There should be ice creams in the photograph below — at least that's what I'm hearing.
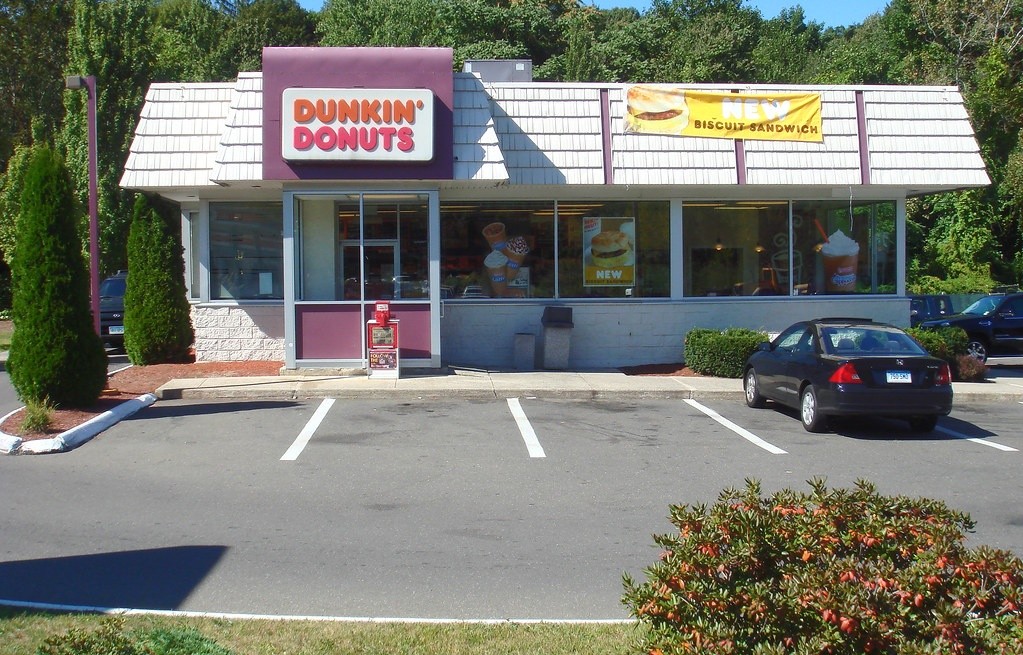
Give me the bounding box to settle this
[482,222,529,295]
[820,229,860,293]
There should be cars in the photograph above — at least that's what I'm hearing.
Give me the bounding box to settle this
[917,291,1023,370]
[90,269,129,345]
[743,316,954,434]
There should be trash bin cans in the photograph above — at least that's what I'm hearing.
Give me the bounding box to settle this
[513,332,536,370]
[541,306,575,370]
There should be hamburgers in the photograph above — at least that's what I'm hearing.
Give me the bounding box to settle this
[628,86,689,135]
[591,230,631,268]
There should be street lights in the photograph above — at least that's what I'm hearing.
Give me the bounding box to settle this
[66,76,101,337]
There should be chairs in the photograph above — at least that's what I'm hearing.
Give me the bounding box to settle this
[887,341,900,352]
[860,336,878,351]
[839,338,854,350]
